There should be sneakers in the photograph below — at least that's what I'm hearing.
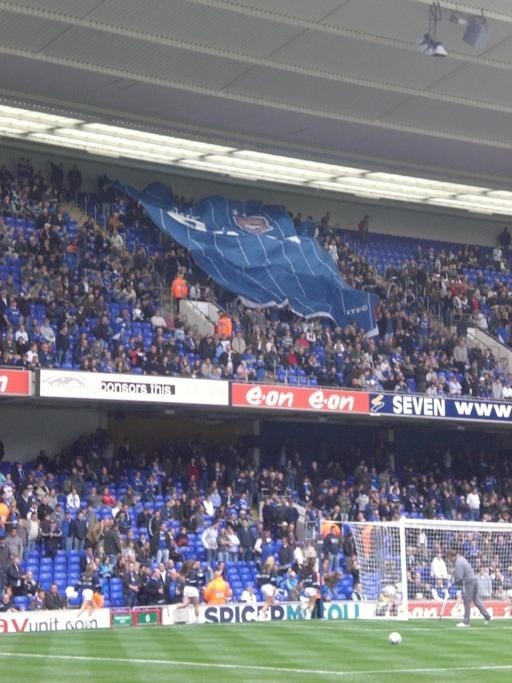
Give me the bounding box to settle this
[455,617,493,629]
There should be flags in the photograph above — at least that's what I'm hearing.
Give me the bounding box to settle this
[112,181,380,340]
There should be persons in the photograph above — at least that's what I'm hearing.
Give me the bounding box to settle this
[0,427,512,629]
[0,155,512,401]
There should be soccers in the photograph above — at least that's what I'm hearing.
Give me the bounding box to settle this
[388,632,402,645]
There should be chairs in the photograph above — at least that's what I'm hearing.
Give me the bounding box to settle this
[3,433,509,609]
[2,190,511,399]
